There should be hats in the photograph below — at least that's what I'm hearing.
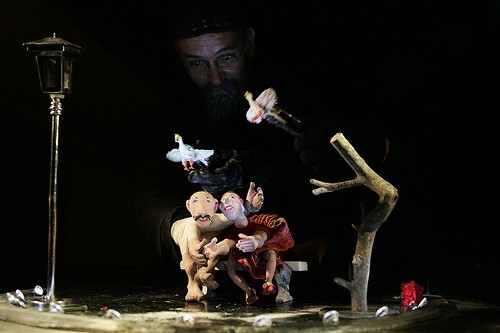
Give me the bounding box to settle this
[174,0,241,39]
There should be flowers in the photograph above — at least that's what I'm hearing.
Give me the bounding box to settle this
[401,283,425,308]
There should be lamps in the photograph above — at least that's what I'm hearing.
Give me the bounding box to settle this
[22,32,82,94]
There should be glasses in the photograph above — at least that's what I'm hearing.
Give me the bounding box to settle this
[184,42,246,73]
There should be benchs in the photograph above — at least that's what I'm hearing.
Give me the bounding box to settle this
[180,261,308,303]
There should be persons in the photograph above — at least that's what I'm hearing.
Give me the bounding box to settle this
[171,182,264,300]
[203,191,294,304]
[109,7,352,295]
